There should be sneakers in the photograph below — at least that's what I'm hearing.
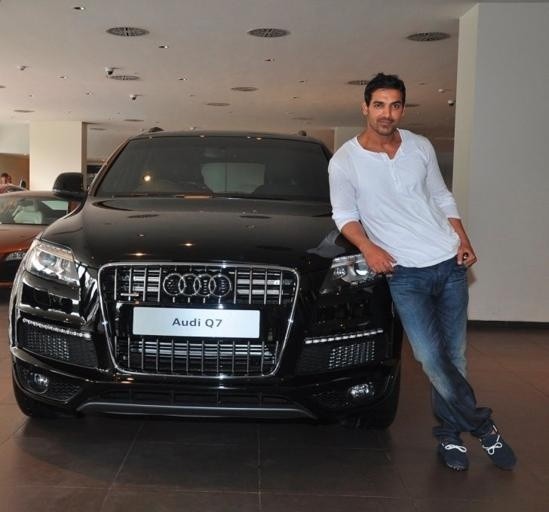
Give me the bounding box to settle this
[438,436,469,471]
[479,418,517,470]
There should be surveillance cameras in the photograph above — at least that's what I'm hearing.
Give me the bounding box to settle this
[448,99,454,106]
[130,94,136,100]
[104,66,114,77]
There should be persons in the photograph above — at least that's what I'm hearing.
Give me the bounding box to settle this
[0,172,15,223]
[327,72,518,472]
